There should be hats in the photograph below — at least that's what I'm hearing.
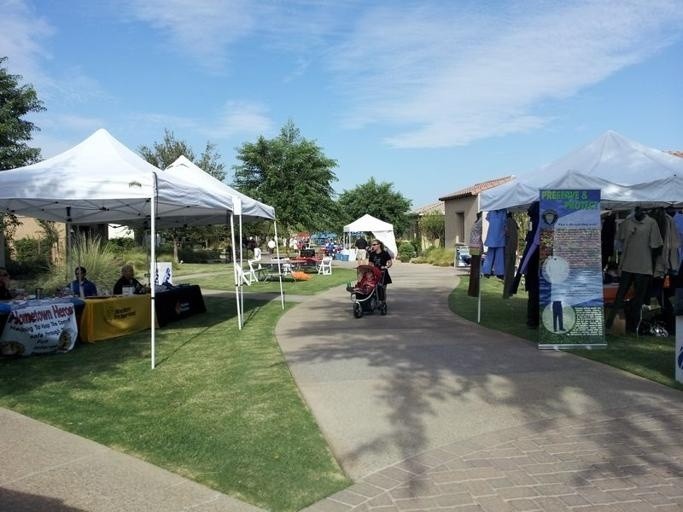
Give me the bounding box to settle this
[544,210,556,215]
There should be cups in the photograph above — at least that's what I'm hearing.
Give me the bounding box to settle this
[34,288,44,299]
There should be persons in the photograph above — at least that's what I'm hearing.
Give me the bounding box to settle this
[236,235,335,257]
[369,239,393,313]
[552,300,566,331]
[162,268,171,286]
[355,235,368,266]
[113,265,146,294]
[0,268,29,299]
[67,266,97,296]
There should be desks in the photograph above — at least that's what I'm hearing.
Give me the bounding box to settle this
[0,283,206,354]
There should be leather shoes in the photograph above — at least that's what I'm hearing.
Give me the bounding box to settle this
[554,328,566,332]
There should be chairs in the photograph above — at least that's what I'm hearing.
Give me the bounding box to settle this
[236,248,333,286]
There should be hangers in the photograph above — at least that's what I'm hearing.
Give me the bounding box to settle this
[505,207,513,219]
[634,201,677,222]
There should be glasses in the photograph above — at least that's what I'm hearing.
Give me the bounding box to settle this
[373,244,379,246]
[75,273,80,275]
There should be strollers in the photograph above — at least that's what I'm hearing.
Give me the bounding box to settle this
[346,265,391,318]
